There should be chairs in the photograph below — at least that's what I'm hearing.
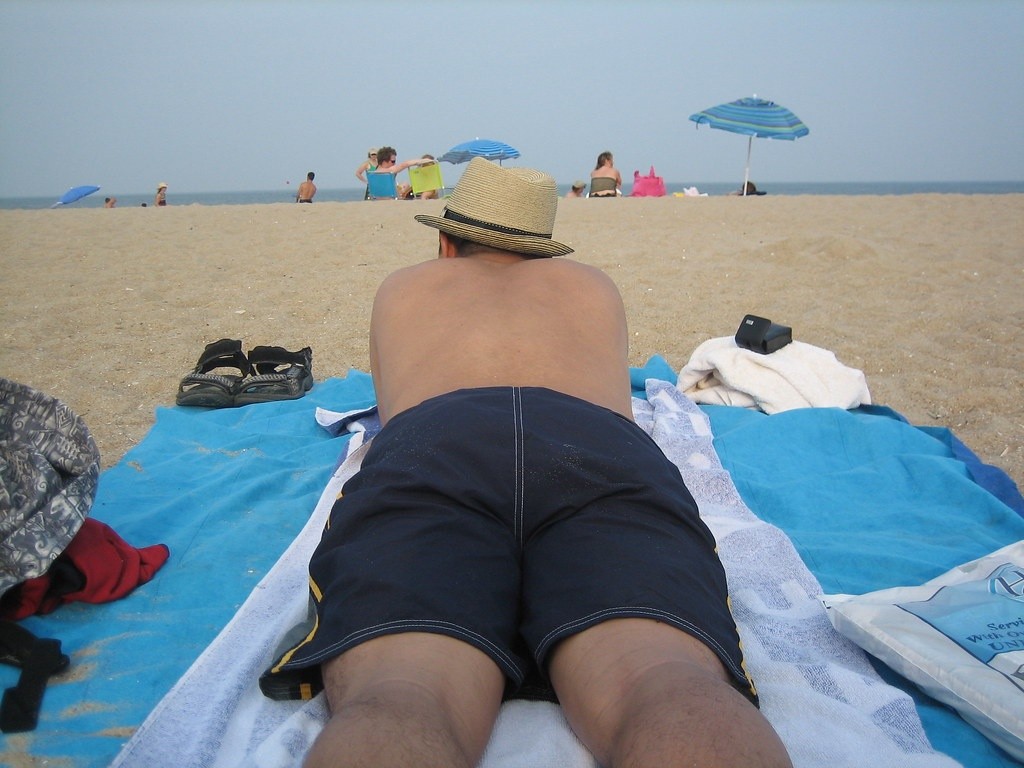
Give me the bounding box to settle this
[366,170,397,199]
[408,160,445,200]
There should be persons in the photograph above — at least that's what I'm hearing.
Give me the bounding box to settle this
[566,180,586,198]
[355,147,378,200]
[104,197,116,208]
[156,183,168,206]
[742,181,756,193]
[375,147,434,200]
[259,153,793,768]
[590,151,622,197]
[403,155,438,200]
[297,171,316,203]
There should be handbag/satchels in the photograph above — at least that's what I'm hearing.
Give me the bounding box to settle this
[631,165,666,197]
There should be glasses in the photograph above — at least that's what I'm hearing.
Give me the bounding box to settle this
[386,159,396,164]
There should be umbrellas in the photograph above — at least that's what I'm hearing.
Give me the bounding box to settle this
[54,185,100,208]
[690,96,807,195]
[438,138,519,164]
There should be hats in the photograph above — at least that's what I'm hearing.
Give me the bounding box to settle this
[414,156,575,257]
[369,148,378,155]
[573,180,585,188]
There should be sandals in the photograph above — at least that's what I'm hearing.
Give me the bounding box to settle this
[234,345,313,406]
[176,338,251,406]
